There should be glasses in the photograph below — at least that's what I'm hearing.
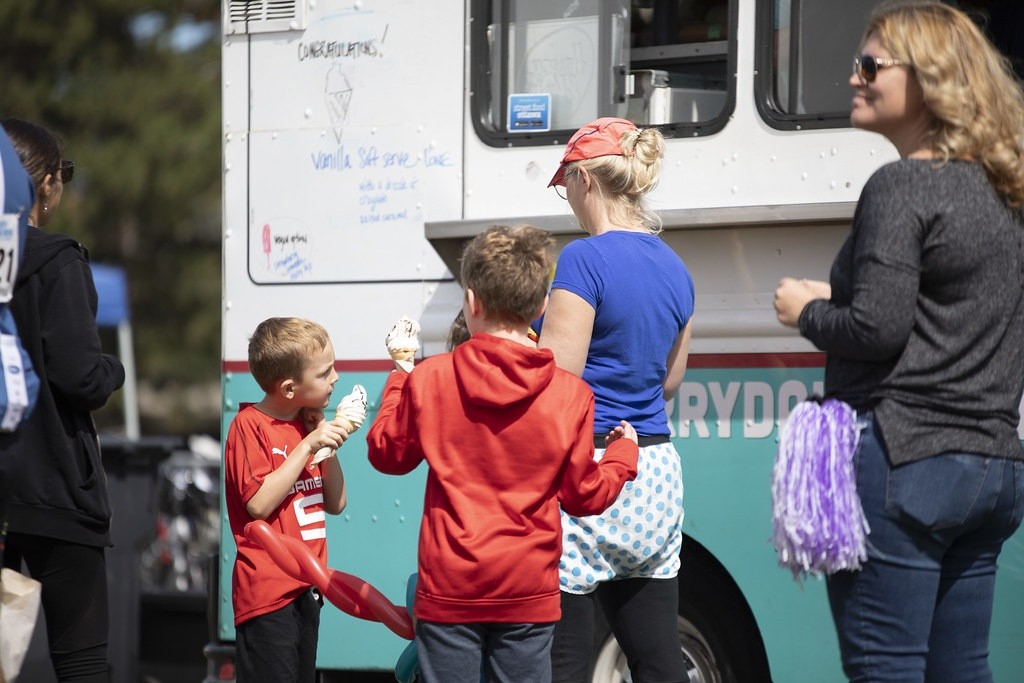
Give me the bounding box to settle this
[853,53,913,81]
[48,160,74,184]
[553,169,574,200]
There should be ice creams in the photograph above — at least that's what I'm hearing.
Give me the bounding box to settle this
[309,384,367,469]
[385,314,420,373]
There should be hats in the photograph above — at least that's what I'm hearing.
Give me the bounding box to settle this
[547,117,639,188]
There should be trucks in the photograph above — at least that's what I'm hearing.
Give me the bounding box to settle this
[216,1,1023,683]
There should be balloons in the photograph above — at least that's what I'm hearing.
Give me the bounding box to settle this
[244,520,418,683]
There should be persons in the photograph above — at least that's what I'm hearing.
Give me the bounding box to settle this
[367,225,638,683]
[225,316,346,683]
[0,117,126,682]
[773,0,1024,683]
[531,117,696,683]
